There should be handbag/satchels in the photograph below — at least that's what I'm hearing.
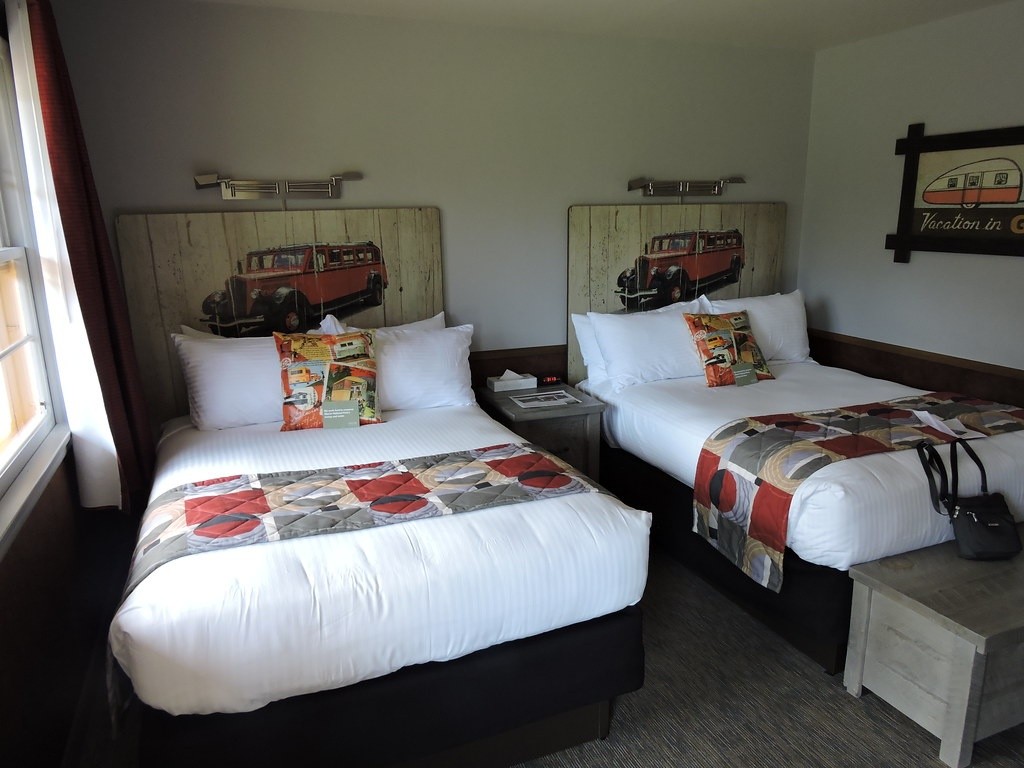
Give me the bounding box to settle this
[916,437,1021,561]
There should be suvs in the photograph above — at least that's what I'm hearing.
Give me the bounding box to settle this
[197,239,389,336]
[613,227,746,311]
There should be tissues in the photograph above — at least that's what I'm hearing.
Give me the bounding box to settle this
[487,369,538,393]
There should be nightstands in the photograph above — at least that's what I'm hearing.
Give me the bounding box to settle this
[483,383,606,484]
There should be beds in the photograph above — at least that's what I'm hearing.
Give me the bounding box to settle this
[563,200,1023,675]
[106,204,655,768]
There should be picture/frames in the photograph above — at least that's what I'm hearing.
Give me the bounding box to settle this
[882,122,1024,265]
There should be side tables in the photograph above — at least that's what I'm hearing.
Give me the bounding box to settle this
[842,522,1024,768]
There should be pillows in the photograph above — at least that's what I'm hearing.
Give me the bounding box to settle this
[710,287,818,365]
[319,314,481,411]
[681,309,775,389]
[587,293,713,392]
[376,310,446,332]
[178,324,226,338]
[170,327,325,432]
[272,329,386,431]
[570,302,687,387]
